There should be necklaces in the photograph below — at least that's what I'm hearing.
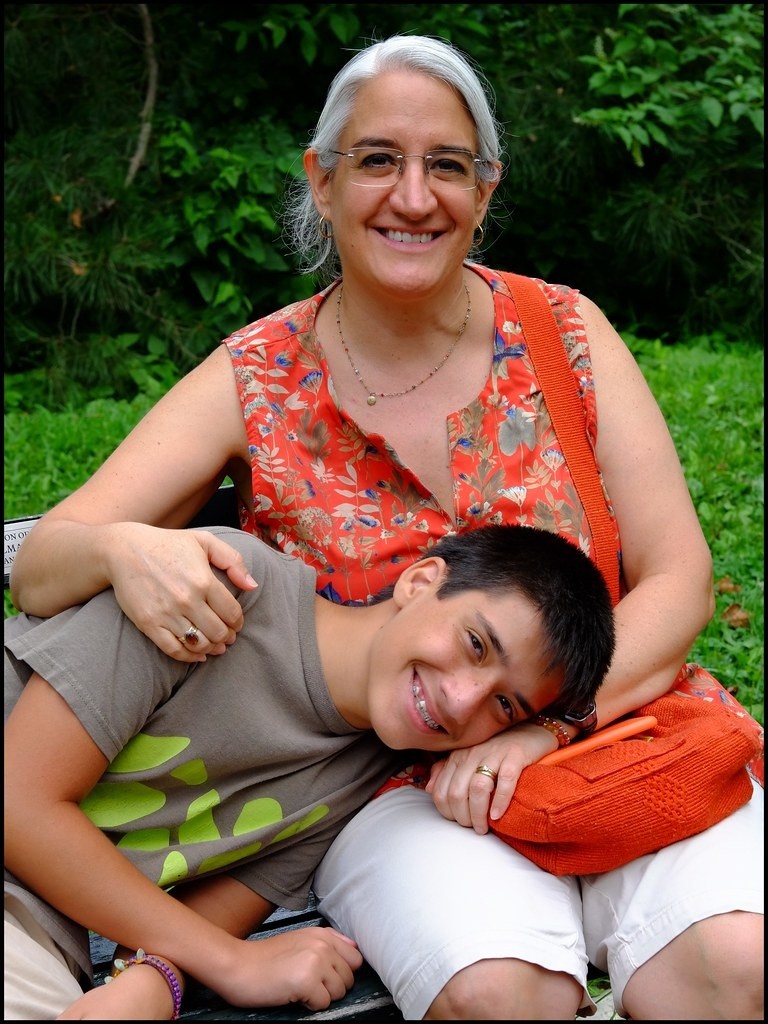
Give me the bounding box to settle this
[337,280,472,404]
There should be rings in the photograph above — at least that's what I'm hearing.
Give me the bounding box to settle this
[177,625,199,646]
[475,766,498,782]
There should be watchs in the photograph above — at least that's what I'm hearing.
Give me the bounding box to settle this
[543,690,598,742]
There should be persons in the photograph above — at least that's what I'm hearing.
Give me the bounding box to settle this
[8,32,765,1022]
[2,525,616,1022]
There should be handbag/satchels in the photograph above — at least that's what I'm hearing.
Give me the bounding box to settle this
[409,658,762,883]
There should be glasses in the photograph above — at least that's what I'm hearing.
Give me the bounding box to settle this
[331,146,491,192]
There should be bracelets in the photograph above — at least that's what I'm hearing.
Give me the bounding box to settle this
[103,945,182,1022]
[530,713,570,749]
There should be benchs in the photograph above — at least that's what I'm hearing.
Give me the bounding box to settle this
[89,880,403,1020]
